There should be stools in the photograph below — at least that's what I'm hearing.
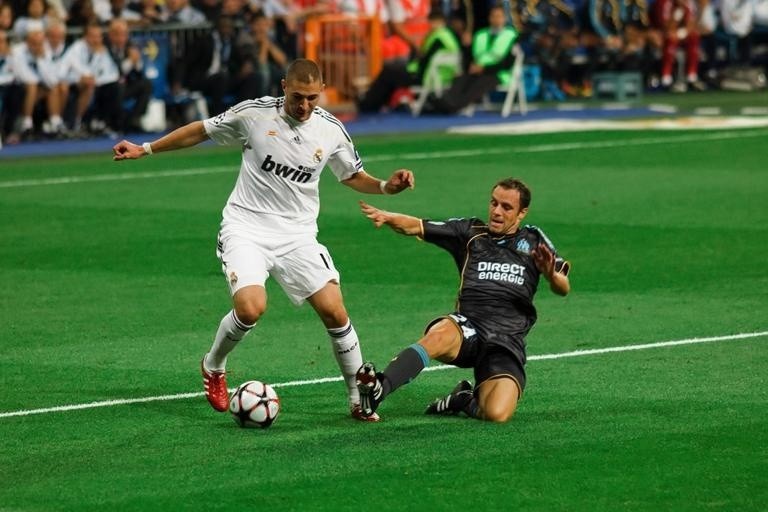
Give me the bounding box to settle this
[593,72,642,103]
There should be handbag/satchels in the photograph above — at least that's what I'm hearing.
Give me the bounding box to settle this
[717,65,767,93]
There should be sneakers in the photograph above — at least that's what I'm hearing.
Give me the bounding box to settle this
[354,91,379,117]
[424,380,475,416]
[200,350,232,413]
[423,93,458,116]
[184,91,211,124]
[665,79,708,95]
[350,361,383,423]
[138,97,168,133]
[541,78,593,102]
[5,124,125,146]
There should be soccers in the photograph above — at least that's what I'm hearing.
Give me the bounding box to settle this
[229,380,279,430]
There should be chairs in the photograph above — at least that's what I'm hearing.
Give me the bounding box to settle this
[398,43,529,120]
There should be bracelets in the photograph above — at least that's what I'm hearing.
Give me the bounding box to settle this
[143,141,152,156]
[378,179,390,195]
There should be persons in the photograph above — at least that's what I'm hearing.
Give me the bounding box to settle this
[112,59,415,423]
[0,0,768,147]
[353,177,572,424]
